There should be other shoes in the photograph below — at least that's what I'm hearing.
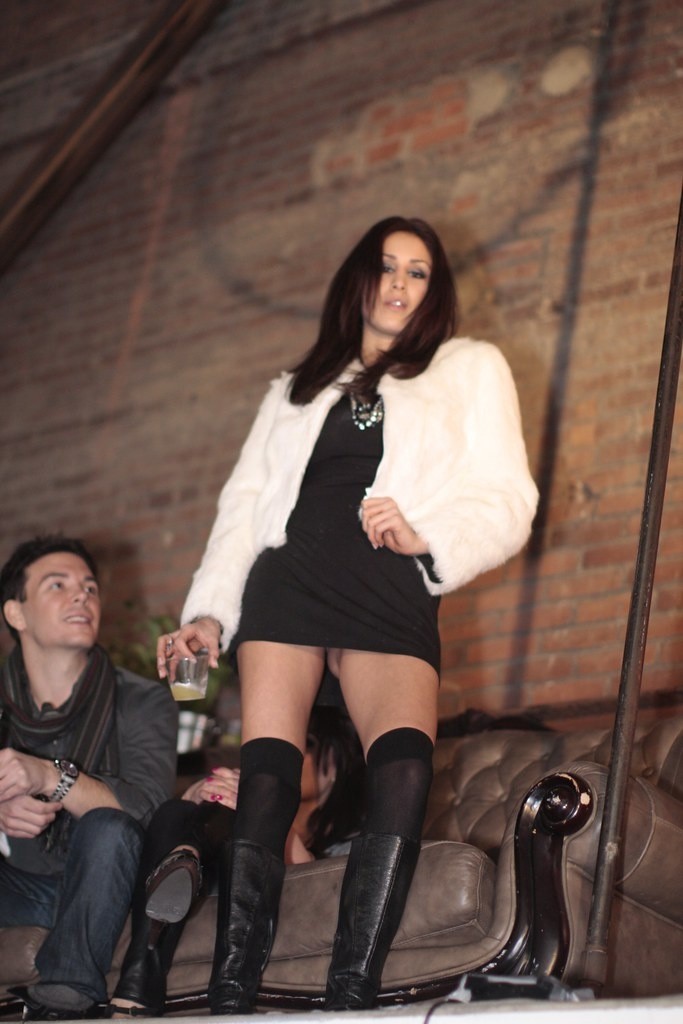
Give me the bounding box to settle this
[6,983,96,1020]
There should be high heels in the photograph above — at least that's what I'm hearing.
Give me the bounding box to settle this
[98,1000,162,1019]
[141,849,210,952]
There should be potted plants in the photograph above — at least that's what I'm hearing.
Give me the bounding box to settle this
[124,609,233,755]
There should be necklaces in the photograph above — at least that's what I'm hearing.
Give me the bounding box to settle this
[350,392,383,430]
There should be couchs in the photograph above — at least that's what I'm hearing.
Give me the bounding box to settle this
[0,708,683,1021]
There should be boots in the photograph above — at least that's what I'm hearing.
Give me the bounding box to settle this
[323,835,423,1011]
[206,840,286,1016]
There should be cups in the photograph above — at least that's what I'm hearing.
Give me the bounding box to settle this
[166,656,209,701]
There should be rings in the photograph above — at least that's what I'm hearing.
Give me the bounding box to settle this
[166,637,172,650]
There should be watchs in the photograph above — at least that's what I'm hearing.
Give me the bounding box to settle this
[48,760,79,802]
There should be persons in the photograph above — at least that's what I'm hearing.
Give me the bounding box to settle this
[110,706,367,1019]
[0,534,178,1020]
[157,215,540,1014]
